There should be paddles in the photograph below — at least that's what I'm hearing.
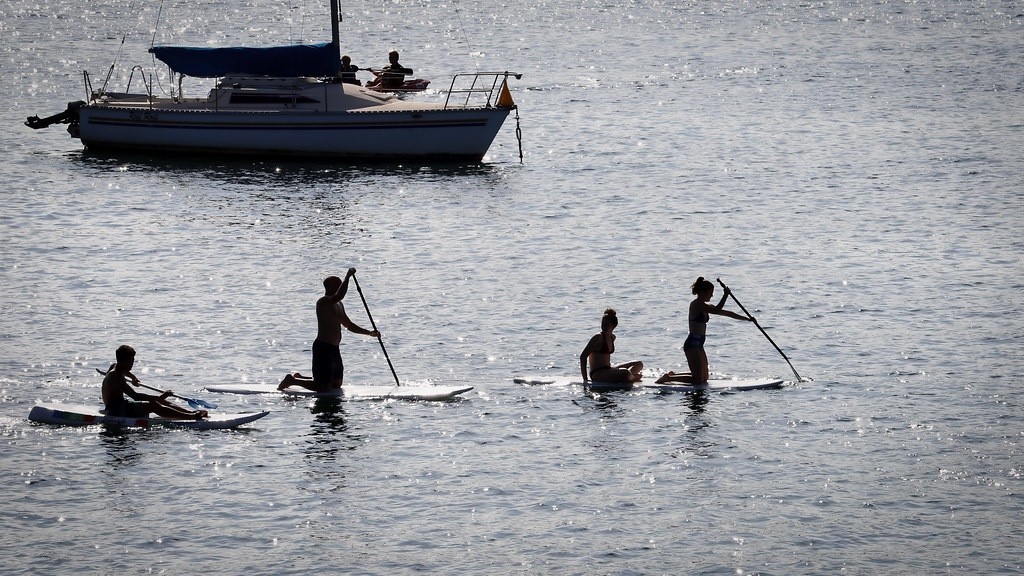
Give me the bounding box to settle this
[716,277,802,381]
[95,367,220,411]
[352,273,399,386]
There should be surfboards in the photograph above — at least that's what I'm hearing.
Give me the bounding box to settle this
[204,384,475,400]
[512,375,784,390]
[28,403,270,429]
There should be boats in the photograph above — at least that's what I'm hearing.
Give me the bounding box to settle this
[24,0,524,164]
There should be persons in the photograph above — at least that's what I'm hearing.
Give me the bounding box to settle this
[340,55,362,85]
[655,277,757,384]
[366,51,413,88]
[276,268,381,392]
[101,345,209,419]
[579,309,643,386]
